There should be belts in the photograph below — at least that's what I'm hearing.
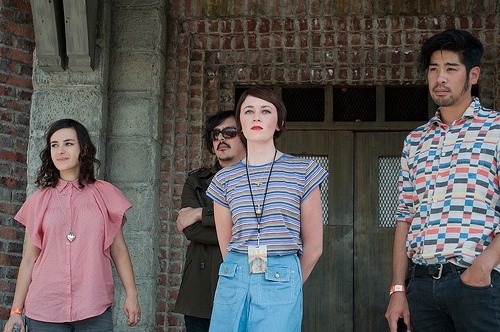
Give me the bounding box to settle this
[409,262,465,278]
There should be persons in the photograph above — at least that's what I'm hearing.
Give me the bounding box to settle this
[384,30,500,332]
[4,118,141,332]
[172,111,249,332]
[209,88,330,332]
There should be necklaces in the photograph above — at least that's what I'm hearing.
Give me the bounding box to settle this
[247,155,272,186]
[54,188,82,242]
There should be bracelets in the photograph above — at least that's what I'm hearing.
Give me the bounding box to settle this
[10,308,23,315]
[389,284,406,295]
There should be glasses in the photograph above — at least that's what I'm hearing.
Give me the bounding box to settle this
[209,127,237,142]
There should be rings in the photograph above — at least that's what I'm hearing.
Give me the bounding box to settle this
[137,317,140,319]
